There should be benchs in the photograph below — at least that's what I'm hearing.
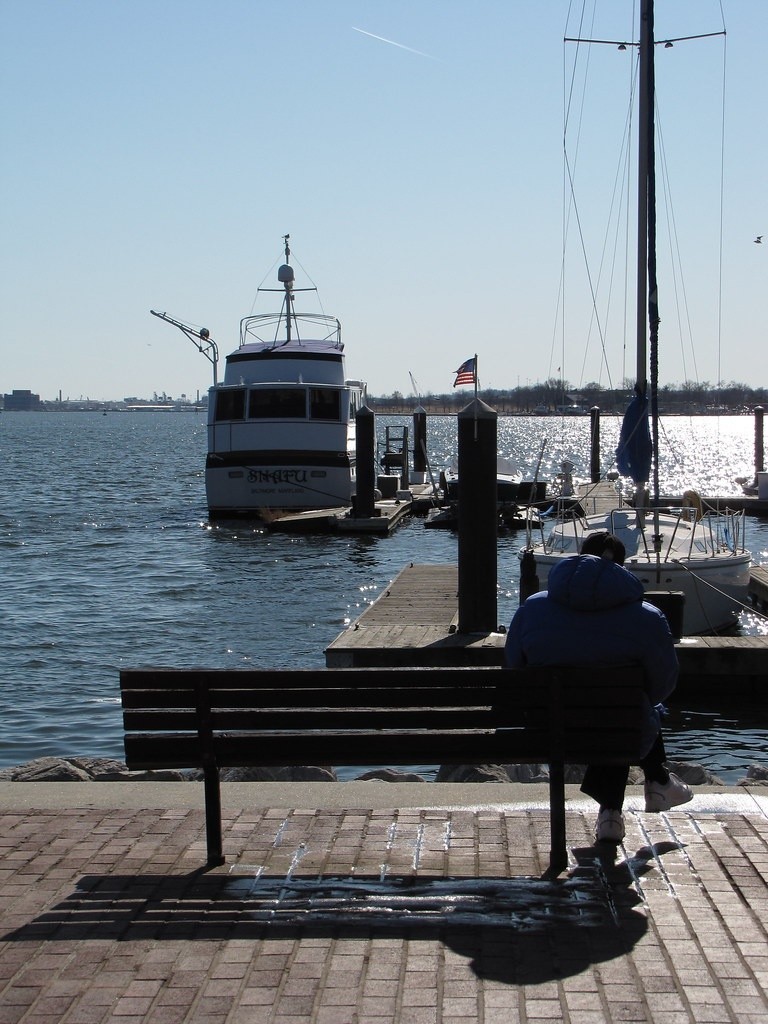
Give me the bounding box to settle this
[119,666,603,884]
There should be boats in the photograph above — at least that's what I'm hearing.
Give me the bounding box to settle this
[146,233,377,520]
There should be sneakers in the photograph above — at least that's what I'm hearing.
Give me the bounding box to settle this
[644,772,694,813]
[591,810,625,846]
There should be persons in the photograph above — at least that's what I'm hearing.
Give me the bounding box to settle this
[492,532,694,843]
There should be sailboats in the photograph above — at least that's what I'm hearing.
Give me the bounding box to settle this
[512,1,756,636]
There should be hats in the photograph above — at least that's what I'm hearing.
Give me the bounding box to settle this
[582,531,626,558]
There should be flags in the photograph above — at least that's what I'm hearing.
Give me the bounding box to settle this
[453,359,475,386]
